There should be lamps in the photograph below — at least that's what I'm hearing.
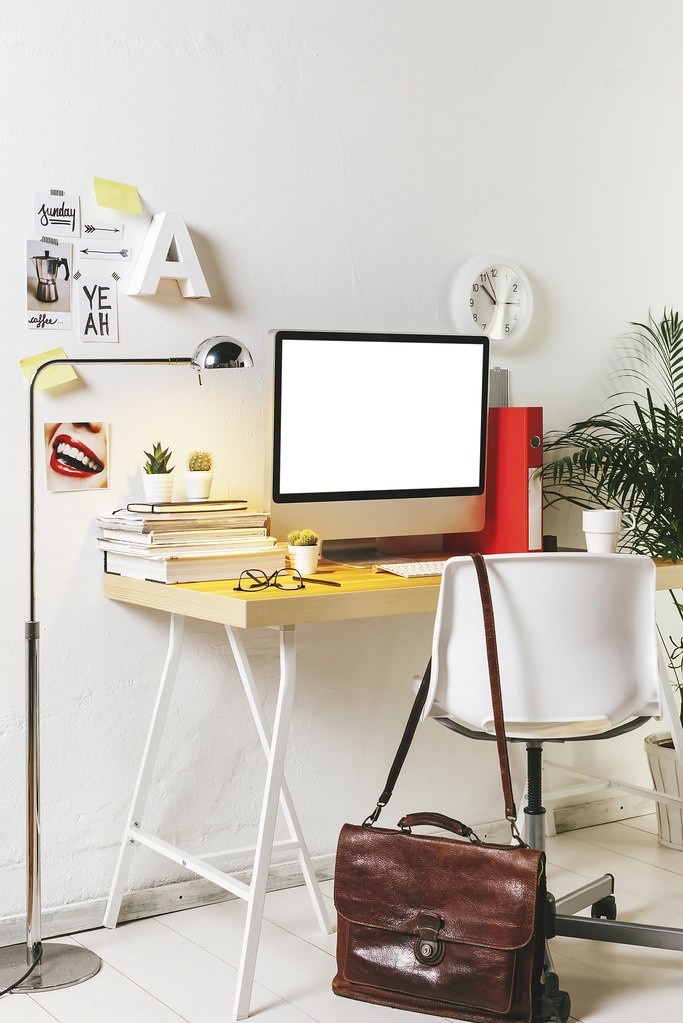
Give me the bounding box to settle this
[1,336,255,995]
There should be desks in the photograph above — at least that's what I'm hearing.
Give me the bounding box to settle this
[104,536,683,1020]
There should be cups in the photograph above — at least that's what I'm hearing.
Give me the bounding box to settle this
[582,509,636,553]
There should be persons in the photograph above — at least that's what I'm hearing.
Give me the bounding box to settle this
[44,423,108,490]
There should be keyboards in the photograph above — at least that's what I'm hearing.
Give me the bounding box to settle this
[372,560,448,577]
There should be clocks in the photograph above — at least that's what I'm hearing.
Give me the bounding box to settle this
[452,254,533,353]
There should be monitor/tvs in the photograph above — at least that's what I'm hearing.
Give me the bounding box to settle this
[264,329,490,569]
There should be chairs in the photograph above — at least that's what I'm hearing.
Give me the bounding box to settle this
[411,550,683,1023]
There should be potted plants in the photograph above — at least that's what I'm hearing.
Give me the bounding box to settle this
[141,440,174,504]
[286,530,320,575]
[529,291,683,852]
[183,447,214,499]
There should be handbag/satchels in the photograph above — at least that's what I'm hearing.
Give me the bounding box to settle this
[333,813,547,1023]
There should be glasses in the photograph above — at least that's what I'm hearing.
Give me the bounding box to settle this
[234,567,305,592]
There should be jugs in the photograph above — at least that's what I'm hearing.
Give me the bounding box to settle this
[30,250,70,302]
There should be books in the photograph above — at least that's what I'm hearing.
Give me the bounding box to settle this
[97,499,288,584]
